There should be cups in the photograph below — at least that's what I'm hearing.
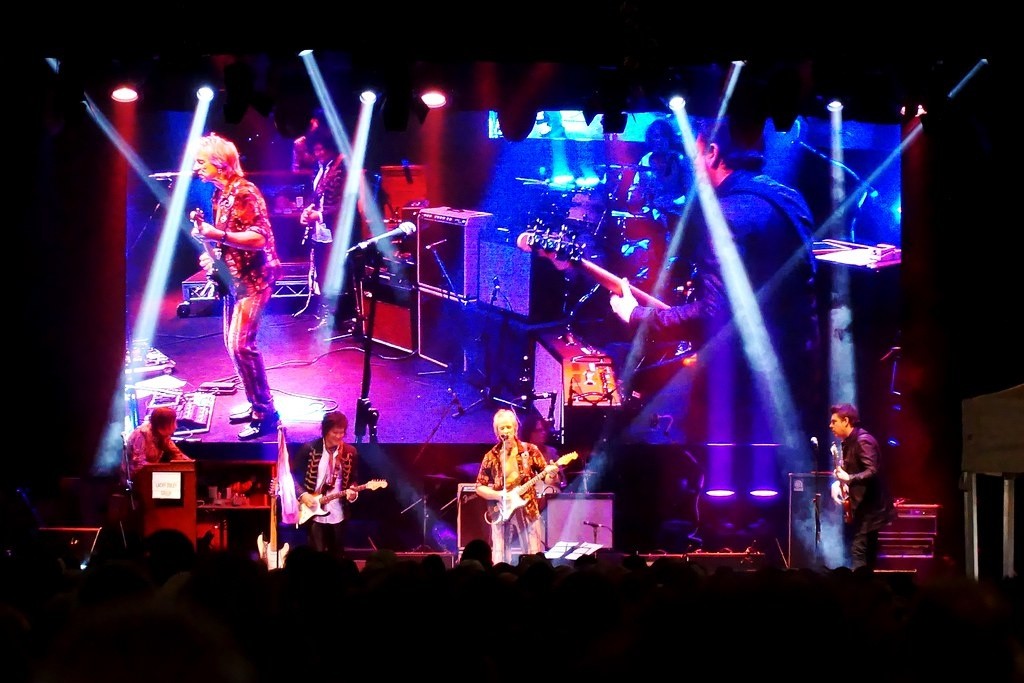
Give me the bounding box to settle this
[209,487,217,500]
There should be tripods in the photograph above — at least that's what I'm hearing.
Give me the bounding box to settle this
[452,289,526,418]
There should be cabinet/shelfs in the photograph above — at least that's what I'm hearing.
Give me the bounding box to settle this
[194,459,278,552]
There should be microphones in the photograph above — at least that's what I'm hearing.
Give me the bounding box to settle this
[150,170,200,180]
[584,521,604,527]
[426,238,448,249]
[493,275,501,290]
[520,392,553,401]
[345,222,417,254]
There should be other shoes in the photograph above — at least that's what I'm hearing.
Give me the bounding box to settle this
[238,411,280,439]
[230,406,252,421]
[308,308,340,333]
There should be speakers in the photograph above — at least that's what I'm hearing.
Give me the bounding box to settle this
[545,492,615,552]
[789,472,851,569]
[359,205,624,449]
[37,525,115,564]
[457,483,522,551]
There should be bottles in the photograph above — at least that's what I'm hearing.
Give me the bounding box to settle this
[233,493,246,505]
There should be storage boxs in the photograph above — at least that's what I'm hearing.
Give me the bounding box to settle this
[417,288,478,373]
[457,484,522,568]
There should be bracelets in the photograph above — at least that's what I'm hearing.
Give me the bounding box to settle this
[549,476,557,479]
[220,232,226,243]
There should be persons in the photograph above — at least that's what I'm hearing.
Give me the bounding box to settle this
[290,118,324,170]
[830,404,897,566]
[301,130,356,332]
[291,412,358,551]
[0,531,1024,683]
[121,408,192,498]
[191,136,283,439]
[610,118,816,442]
[628,120,689,227]
[475,409,559,565]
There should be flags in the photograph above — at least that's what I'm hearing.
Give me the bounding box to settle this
[277,429,297,524]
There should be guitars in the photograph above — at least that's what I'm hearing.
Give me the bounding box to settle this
[184,207,233,301]
[516,218,673,315]
[295,477,389,530]
[830,440,852,524]
[488,451,579,526]
[299,165,340,247]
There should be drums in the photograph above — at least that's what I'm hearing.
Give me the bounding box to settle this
[532,189,613,238]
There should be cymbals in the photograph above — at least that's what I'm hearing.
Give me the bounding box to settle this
[589,160,662,172]
[421,472,455,481]
[567,471,600,475]
